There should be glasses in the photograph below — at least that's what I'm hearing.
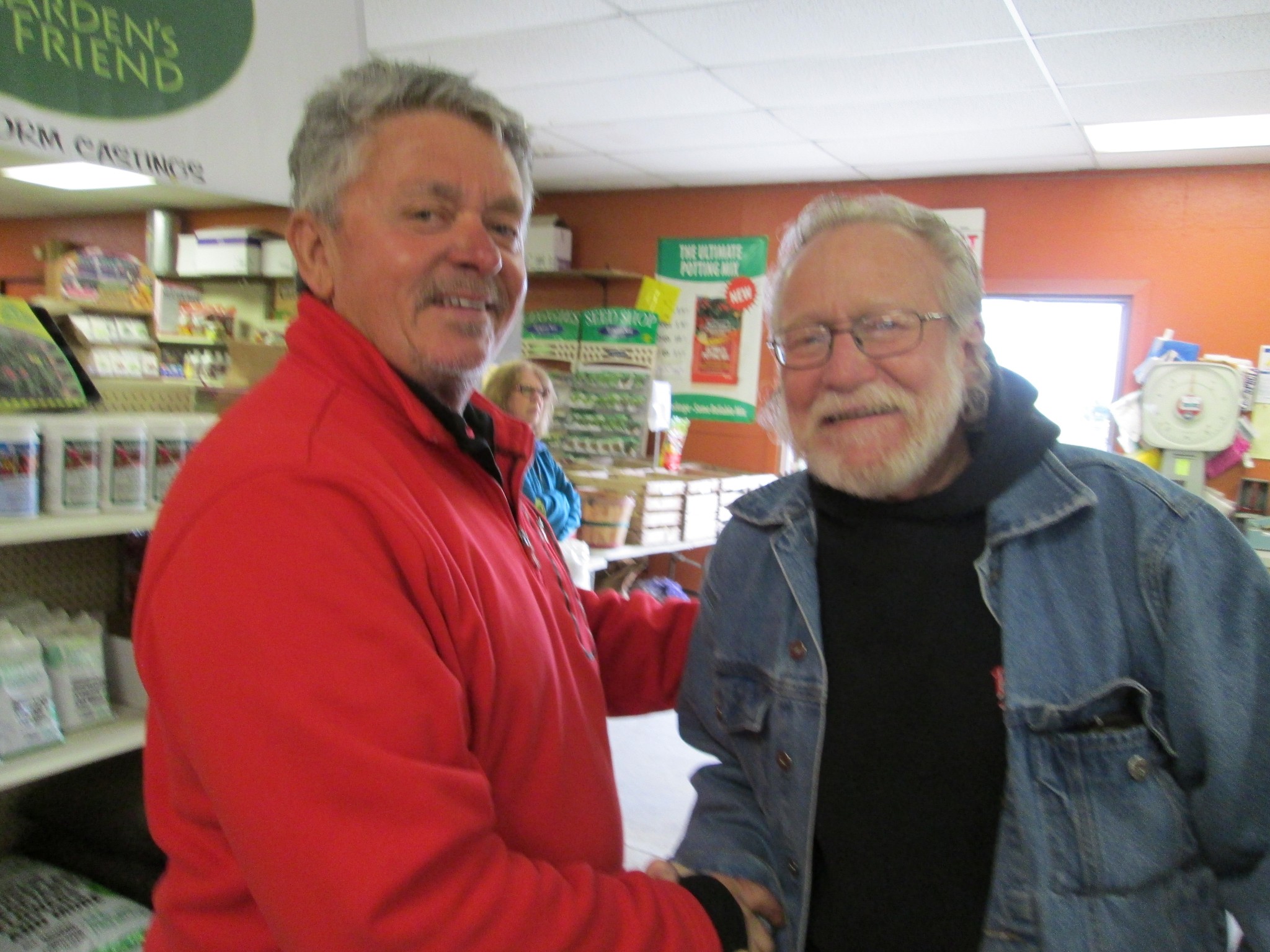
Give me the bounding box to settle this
[765,306,962,369]
[509,383,547,401]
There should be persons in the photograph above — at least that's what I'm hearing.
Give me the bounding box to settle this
[644,190,1270,952]
[129,62,784,952]
[481,360,582,546]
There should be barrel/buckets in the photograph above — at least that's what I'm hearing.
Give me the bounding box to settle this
[580,492,634,549]
[0,412,219,520]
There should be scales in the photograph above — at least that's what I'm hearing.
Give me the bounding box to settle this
[1141,362,1242,496]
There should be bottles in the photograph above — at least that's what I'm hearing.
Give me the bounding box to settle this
[183,349,229,387]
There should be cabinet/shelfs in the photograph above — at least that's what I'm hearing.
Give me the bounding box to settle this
[556,366,650,462]
[0,498,169,799]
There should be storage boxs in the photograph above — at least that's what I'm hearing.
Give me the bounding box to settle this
[178,215,573,271]
